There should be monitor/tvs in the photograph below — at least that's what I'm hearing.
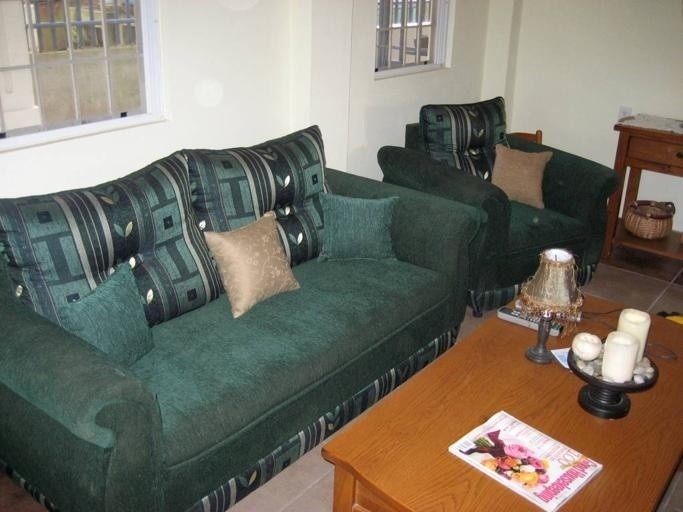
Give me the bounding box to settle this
[447,411,602,512]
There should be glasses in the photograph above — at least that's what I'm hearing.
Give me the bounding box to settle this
[518,247,586,364]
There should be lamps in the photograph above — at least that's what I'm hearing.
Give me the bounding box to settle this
[378,94,621,321]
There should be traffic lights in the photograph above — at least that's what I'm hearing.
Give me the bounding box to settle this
[200,209,303,322]
[54,263,156,371]
[315,187,401,262]
[489,142,557,211]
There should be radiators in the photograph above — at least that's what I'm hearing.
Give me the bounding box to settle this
[514,299,583,322]
[497,307,564,337]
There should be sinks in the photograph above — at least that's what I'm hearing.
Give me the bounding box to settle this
[0,126,493,512]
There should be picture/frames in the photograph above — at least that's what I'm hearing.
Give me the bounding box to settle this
[600,115,683,264]
